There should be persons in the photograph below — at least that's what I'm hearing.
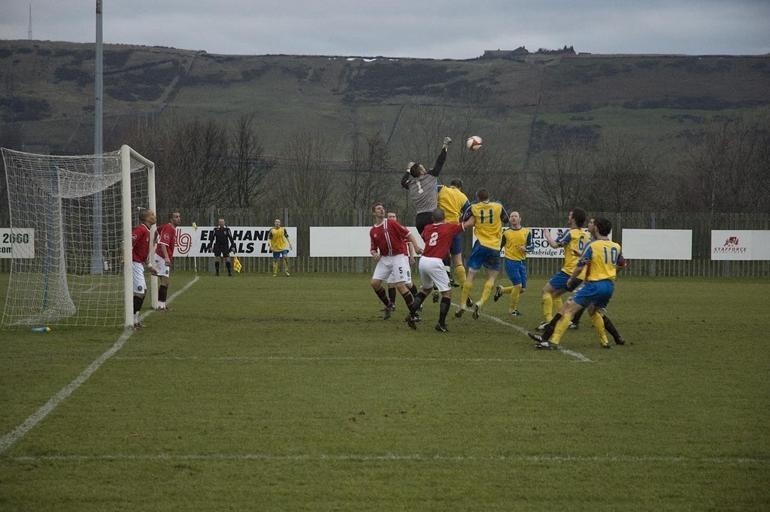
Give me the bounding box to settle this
[401,137,459,302]
[268,219,293,278]
[151,211,181,312]
[132,207,157,329]
[369,178,473,332]
[455,187,510,320]
[495,210,534,316]
[529,208,626,351]
[207,217,236,277]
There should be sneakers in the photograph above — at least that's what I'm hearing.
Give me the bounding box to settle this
[134,322,145,330]
[380,280,624,351]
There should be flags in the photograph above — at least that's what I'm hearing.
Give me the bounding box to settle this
[233,255,243,274]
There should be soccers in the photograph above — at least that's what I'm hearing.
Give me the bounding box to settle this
[466,135,481,151]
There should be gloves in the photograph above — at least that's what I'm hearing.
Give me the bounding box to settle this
[443,136,452,144]
[406,161,415,174]
